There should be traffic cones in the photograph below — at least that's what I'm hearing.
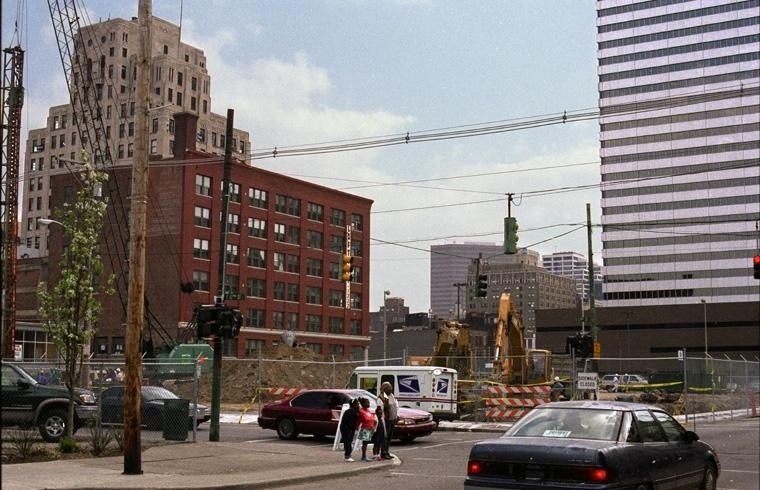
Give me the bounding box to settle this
[747,391,760,418]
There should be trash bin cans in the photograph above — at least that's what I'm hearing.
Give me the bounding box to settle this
[163,399,192,441]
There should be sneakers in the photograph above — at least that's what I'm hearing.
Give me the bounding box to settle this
[346,458,354,461]
[362,456,373,461]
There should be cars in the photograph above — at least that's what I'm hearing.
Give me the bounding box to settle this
[257,388,437,444]
[82,385,210,431]
[726,381,760,394]
[464,399,721,490]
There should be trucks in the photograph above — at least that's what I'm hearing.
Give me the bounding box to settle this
[343,365,458,432]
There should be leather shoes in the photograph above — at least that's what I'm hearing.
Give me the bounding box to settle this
[381,453,394,459]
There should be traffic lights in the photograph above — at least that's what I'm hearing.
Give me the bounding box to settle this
[197,308,215,338]
[477,274,487,298]
[339,253,354,282]
[217,308,245,339]
[567,333,594,368]
[503,215,520,255]
[753,255,760,280]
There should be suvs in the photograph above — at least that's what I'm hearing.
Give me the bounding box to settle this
[0,361,99,443]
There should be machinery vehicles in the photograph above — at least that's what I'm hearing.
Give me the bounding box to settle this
[47,0,214,381]
[473,293,574,401]
[406,321,487,420]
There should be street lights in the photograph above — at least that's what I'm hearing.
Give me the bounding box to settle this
[621,308,636,371]
[453,282,468,321]
[383,290,390,365]
[701,298,709,374]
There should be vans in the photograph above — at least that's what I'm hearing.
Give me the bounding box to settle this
[598,374,648,393]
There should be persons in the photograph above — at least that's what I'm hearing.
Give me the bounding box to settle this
[611,373,619,392]
[358,397,375,461]
[622,373,630,393]
[377,381,399,460]
[551,376,564,394]
[373,404,387,461]
[340,398,361,463]
